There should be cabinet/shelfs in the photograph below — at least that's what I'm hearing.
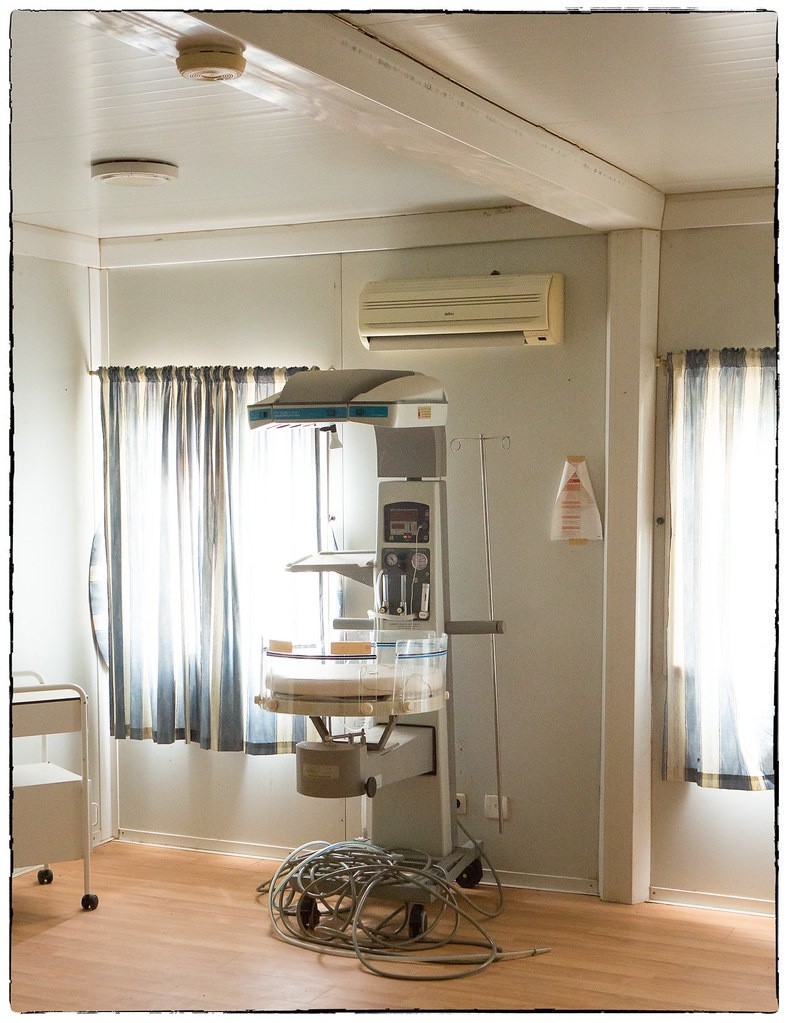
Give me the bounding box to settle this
[13,669,100,911]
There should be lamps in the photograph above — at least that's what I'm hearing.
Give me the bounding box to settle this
[90,161,178,186]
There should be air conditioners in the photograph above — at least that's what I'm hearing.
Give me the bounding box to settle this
[358,272,564,352]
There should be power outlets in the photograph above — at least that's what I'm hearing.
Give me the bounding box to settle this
[457,794,467,815]
[485,795,508,821]
